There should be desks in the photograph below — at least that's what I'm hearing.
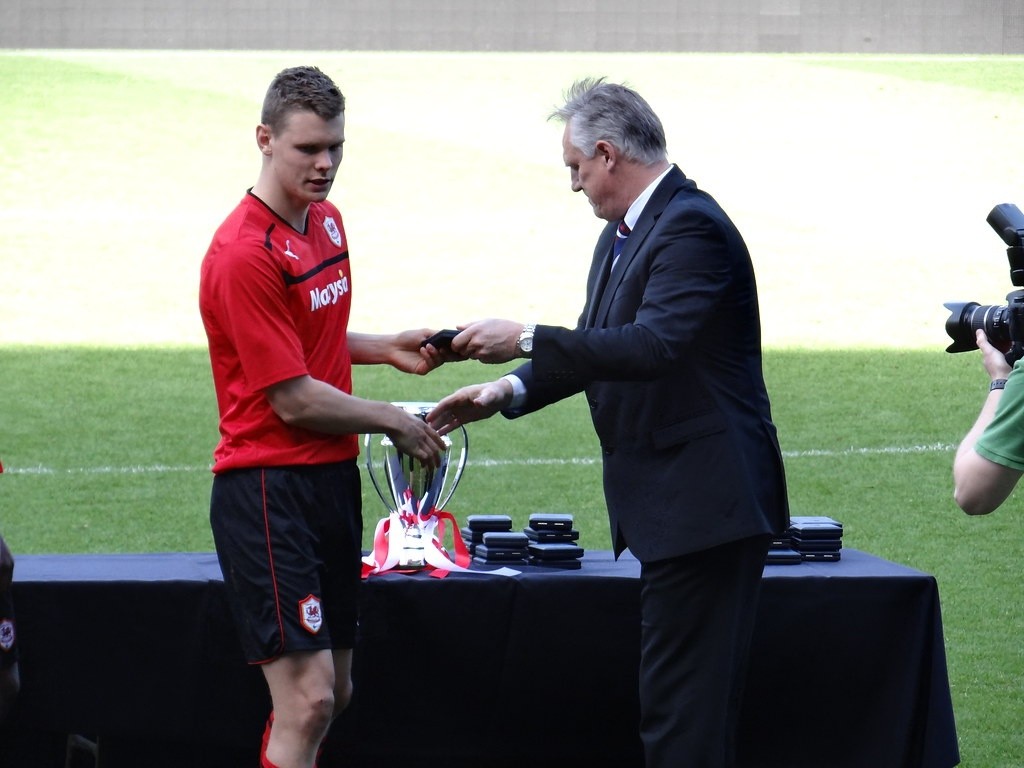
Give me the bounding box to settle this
[9,545,957,766]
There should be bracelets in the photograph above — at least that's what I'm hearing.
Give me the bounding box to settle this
[990,379,1007,389]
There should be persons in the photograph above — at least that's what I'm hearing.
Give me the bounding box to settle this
[427,78,791,768]
[199,66,445,768]
[951,329,1024,516]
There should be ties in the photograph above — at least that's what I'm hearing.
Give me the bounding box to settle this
[611,218,630,272]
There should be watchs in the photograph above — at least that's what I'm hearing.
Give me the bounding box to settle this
[516,325,536,357]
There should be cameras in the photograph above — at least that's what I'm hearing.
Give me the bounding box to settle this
[942,203,1024,365]
